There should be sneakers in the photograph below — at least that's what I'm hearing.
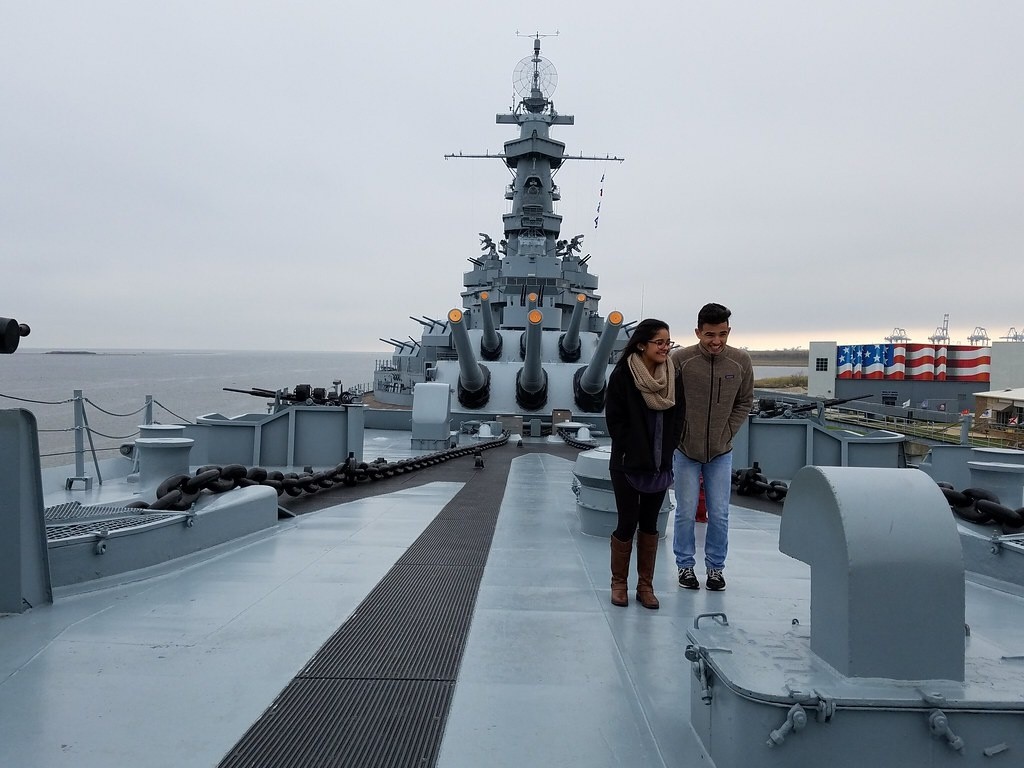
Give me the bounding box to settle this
[705,570,726,591]
[677,568,700,589]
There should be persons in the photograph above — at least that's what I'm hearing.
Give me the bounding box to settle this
[605,319,688,608]
[669,303,755,590]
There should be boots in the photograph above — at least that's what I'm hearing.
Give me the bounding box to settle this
[609,532,634,606]
[636,529,660,609]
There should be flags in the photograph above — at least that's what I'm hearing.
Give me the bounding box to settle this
[937,403,946,411]
[902,399,911,409]
[1009,417,1017,425]
[979,410,992,418]
[836,345,991,382]
[921,401,928,409]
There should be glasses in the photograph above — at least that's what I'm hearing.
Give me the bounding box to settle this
[647,339,675,349]
